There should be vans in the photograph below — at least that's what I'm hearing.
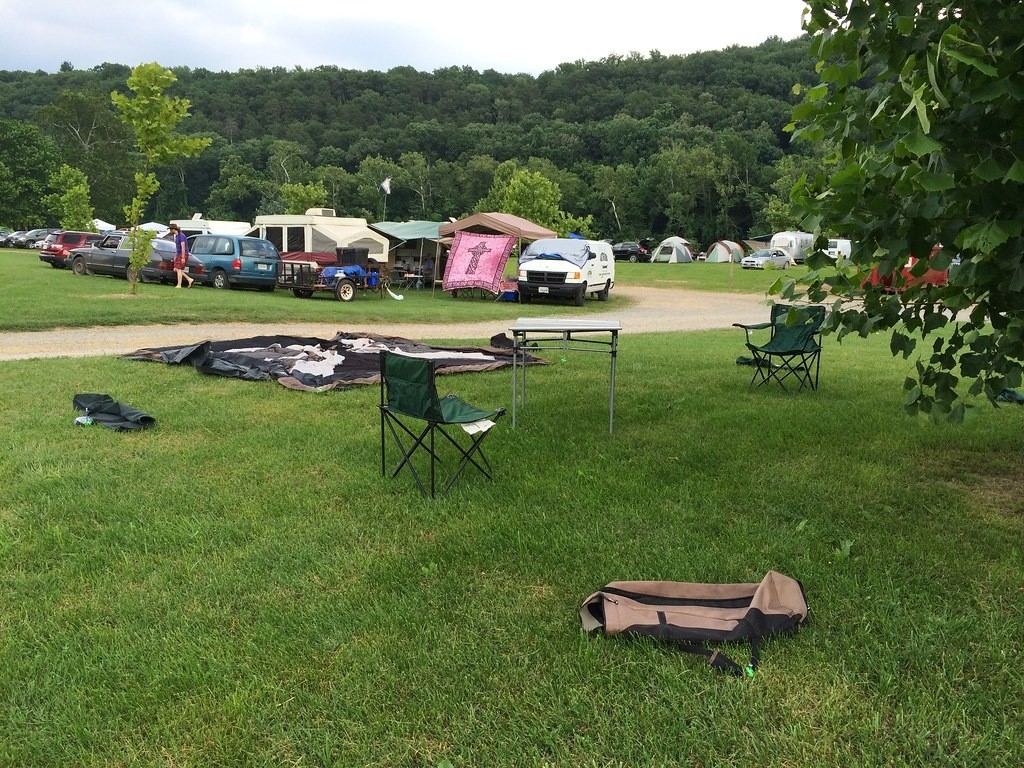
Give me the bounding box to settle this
[517,240,615,305]
[822,239,851,261]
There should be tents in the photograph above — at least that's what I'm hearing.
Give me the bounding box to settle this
[86,218,116,230]
[704,240,744,263]
[650,235,693,263]
[432,211,557,300]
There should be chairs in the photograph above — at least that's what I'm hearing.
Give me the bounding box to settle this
[376,349,507,502]
[732,304,826,396]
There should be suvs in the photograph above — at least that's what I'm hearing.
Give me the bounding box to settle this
[39,229,118,269]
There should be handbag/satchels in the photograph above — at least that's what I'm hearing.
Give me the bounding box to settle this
[578,571,808,677]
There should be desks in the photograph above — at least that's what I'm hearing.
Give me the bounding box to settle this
[507,317,622,433]
[404,275,423,292]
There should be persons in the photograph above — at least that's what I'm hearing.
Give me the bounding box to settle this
[167,224,194,288]
[423,252,434,271]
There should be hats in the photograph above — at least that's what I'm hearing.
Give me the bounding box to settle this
[167,224,180,230]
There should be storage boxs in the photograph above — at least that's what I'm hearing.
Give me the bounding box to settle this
[501,289,517,303]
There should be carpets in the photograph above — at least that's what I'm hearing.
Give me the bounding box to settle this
[120,330,551,393]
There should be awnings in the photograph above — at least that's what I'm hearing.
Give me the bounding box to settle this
[367,221,451,292]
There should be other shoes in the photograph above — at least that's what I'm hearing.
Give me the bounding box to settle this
[188,278,194,288]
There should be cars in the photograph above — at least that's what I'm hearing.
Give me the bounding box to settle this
[741,249,791,270]
[613,237,656,263]
[0,229,55,250]
[156,227,229,252]
[64,232,208,285]
[186,234,283,291]
